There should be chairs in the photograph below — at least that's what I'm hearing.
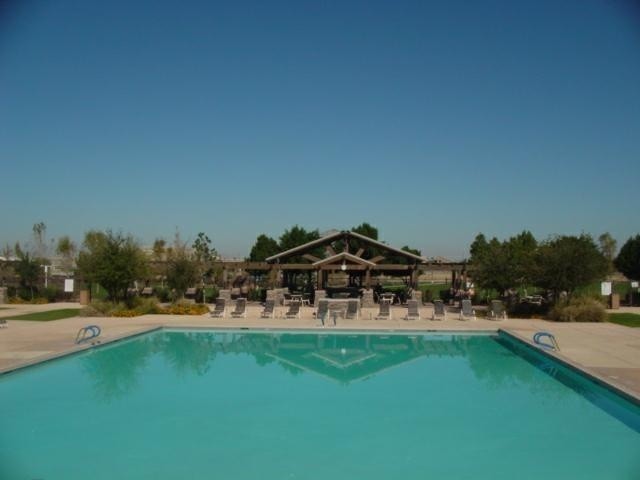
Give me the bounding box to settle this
[205,284,508,321]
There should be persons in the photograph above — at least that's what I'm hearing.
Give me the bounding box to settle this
[375,282,382,291]
[449,286,475,308]
